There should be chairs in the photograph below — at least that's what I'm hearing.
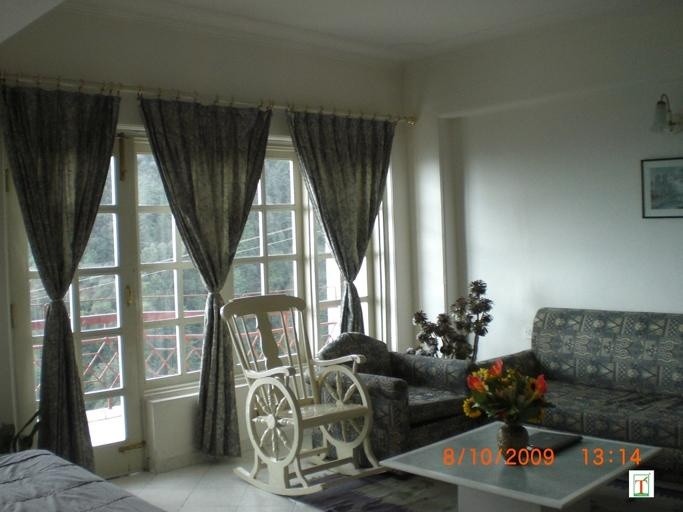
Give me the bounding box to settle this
[220,296,390,497]
[318,330,477,479]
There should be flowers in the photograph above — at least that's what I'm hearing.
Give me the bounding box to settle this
[463,357,555,424]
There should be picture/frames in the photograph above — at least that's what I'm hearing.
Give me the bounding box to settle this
[640,159,681,220]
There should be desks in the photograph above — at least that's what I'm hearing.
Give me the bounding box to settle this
[379,421,662,512]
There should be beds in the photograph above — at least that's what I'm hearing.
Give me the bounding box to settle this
[0,446,165,512]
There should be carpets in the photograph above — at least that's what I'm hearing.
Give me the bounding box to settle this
[284,474,683,511]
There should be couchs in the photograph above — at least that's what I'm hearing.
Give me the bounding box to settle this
[472,303,681,480]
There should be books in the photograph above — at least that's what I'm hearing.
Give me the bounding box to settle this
[525,430,583,458]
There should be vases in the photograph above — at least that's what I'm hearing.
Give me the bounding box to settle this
[498,421,529,467]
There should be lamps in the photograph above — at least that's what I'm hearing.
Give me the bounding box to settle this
[650,95,680,140]
